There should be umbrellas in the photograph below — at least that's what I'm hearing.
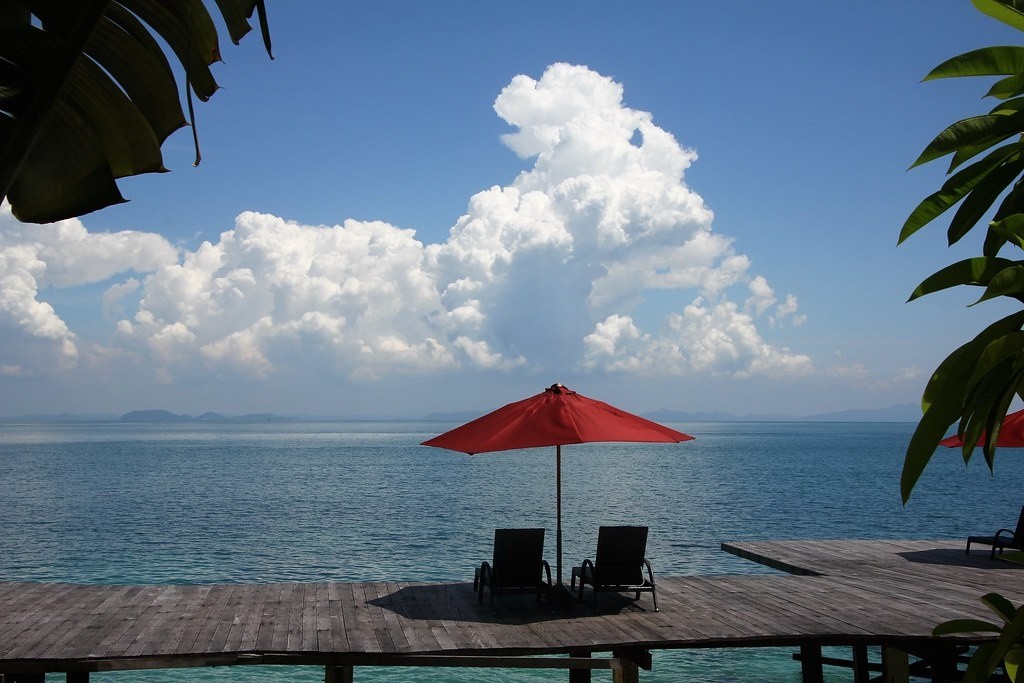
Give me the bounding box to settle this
[420,383,696,584]
[937,407,1024,450]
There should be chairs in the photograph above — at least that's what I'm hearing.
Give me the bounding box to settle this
[572,526,659,612]
[966,506,1024,560]
[474,528,553,616]
